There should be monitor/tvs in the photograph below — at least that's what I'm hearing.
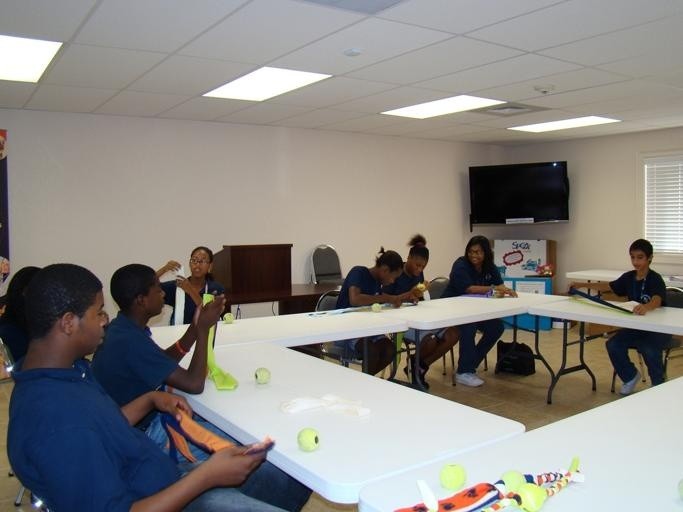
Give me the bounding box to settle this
[467,160,570,226]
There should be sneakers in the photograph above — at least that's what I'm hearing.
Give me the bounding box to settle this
[407,357,429,387]
[403,366,429,389]
[454,371,485,387]
[619,370,641,395]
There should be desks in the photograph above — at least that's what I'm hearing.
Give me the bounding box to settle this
[223,285,343,315]
[567,269,669,296]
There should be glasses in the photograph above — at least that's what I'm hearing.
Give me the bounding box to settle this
[190,258,210,265]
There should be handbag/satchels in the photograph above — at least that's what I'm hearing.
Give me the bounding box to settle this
[496,339,535,376]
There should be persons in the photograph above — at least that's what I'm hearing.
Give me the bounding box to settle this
[440,236,518,388]
[3,262,313,511]
[569,239,665,396]
[336,246,423,377]
[381,233,459,391]
[156,248,228,327]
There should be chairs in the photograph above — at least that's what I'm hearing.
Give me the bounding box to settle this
[0,313,49,512]
[315,277,488,386]
[310,243,342,284]
[611,287,682,394]
